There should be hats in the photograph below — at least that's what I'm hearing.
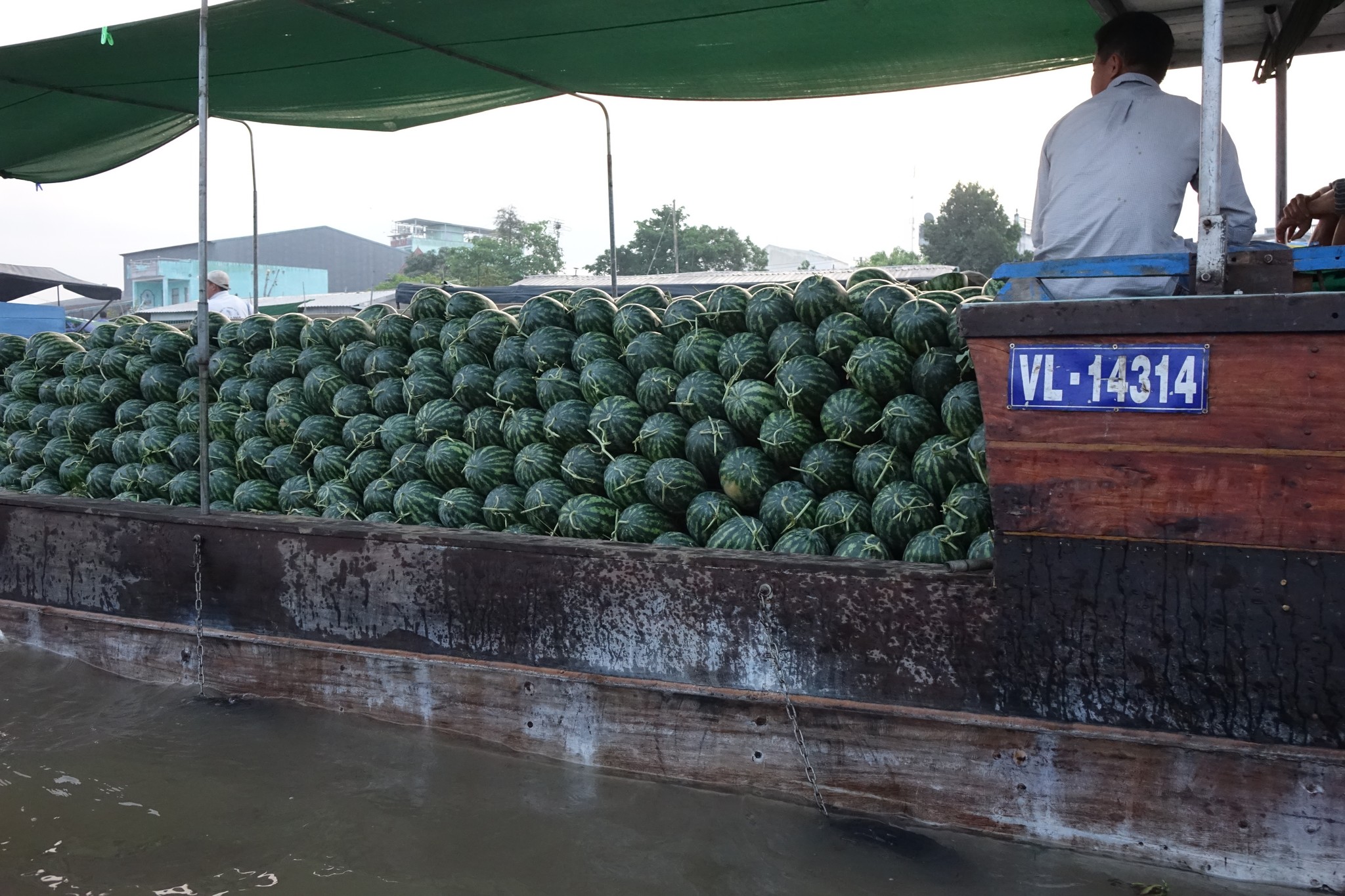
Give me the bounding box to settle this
[196,270,231,290]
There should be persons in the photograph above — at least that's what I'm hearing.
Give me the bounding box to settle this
[1032,12,1258,308]
[1272,176,1345,249]
[196,267,261,324]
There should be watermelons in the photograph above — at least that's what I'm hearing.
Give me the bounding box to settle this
[0,267,1001,565]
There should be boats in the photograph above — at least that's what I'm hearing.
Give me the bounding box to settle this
[0,0,1345,896]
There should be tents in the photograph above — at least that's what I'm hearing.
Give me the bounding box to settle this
[0,0,1345,518]
[0,263,123,335]
